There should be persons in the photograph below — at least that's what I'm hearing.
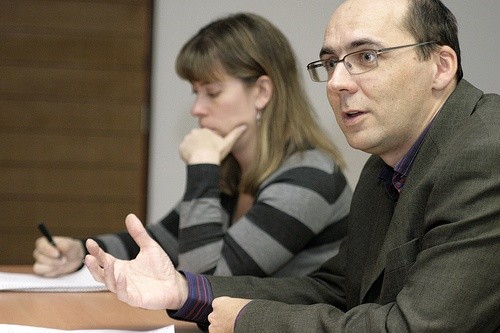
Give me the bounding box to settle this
[83,0,500,333]
[33,12,356,282]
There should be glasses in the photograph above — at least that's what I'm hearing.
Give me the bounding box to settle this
[306,40,437,82]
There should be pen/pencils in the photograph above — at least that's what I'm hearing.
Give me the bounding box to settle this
[37,223,56,247]
